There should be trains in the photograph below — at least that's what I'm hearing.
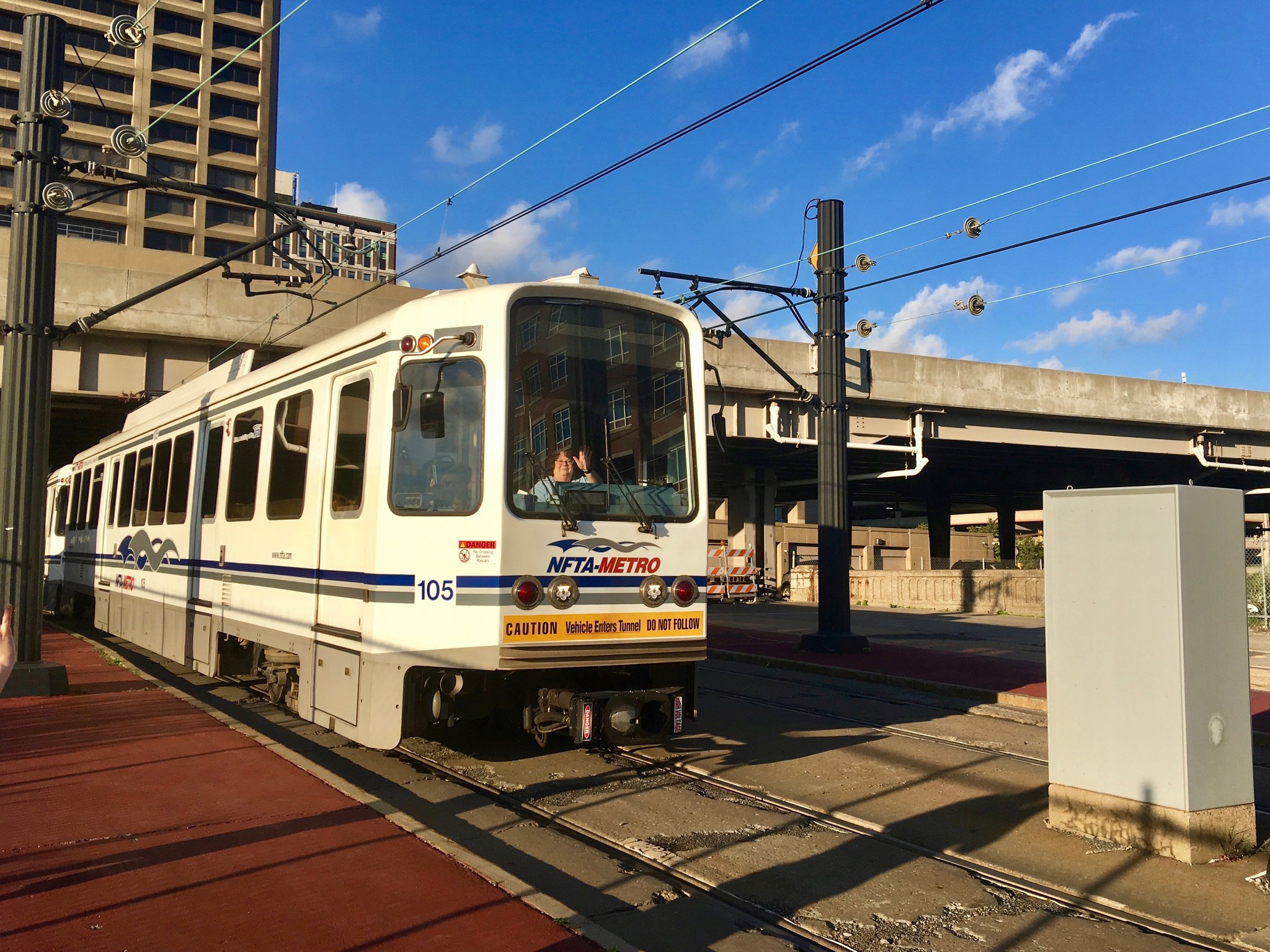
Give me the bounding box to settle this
[45,219,730,753]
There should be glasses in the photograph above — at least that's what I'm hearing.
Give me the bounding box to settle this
[555,459,574,465]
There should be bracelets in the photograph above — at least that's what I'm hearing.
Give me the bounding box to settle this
[583,468,592,474]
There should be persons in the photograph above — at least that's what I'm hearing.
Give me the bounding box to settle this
[0,604,17,693]
[530,444,603,503]
